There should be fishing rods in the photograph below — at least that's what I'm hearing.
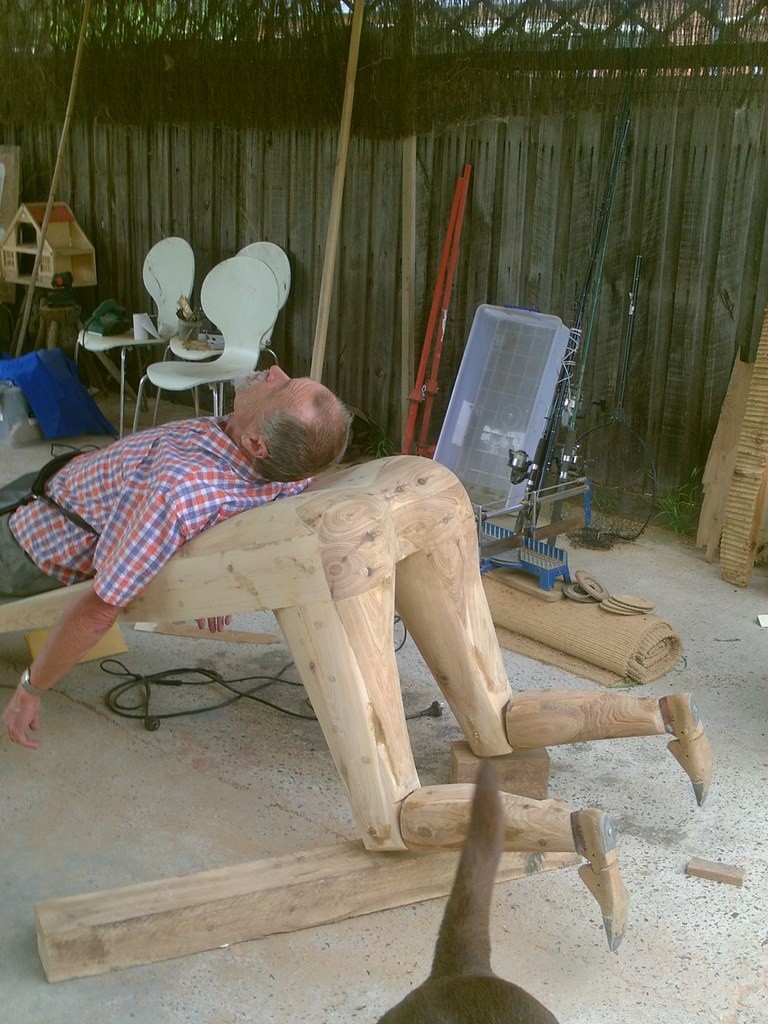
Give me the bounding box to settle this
[507,245,609,547]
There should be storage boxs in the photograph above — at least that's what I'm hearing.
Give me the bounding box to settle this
[178,318,208,351]
[25,621,128,666]
[429,302,572,520]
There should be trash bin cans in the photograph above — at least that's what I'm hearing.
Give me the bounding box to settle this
[0,380,40,444]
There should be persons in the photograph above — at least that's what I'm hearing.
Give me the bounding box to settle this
[0,364,356,749]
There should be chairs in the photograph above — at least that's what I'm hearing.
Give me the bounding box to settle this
[71,236,198,442]
[152,242,292,428]
[132,255,279,433]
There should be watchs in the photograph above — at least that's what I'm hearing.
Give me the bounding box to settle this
[20,668,46,696]
